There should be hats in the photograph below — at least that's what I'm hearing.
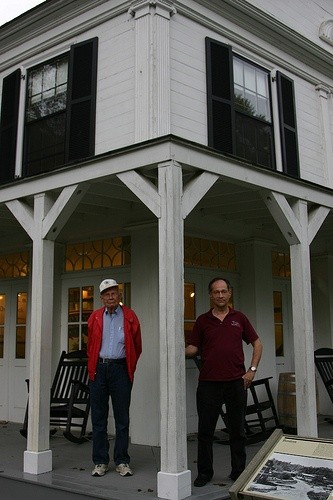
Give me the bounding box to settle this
[98,278,119,293]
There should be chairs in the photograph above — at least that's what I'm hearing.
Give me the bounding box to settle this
[193,350,297,446]
[20,350,91,444]
[314,347,333,404]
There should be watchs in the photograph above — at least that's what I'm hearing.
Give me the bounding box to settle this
[249,366,257,372]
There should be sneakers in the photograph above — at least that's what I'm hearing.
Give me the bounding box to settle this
[116,464,133,477]
[91,463,110,477]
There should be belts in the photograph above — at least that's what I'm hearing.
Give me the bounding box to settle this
[97,358,127,364]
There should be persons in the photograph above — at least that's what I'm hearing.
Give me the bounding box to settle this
[185,278,263,487]
[87,278,142,477]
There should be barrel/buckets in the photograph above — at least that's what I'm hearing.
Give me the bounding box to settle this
[277,372,320,436]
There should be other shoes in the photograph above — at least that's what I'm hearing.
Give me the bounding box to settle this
[194,475,214,487]
[229,475,239,481]
